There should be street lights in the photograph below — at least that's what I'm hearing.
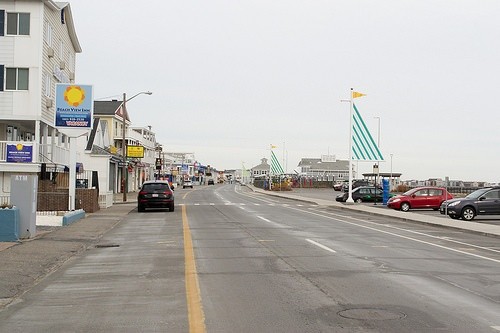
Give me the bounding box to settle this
[115,91,153,202]
[390,152,394,189]
[375,115,381,183]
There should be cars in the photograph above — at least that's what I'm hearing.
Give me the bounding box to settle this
[332,178,384,192]
[335,187,398,203]
[447,187,500,221]
[207,179,214,185]
[183,180,193,189]
[440,188,494,215]
[387,186,453,212]
[137,179,175,212]
